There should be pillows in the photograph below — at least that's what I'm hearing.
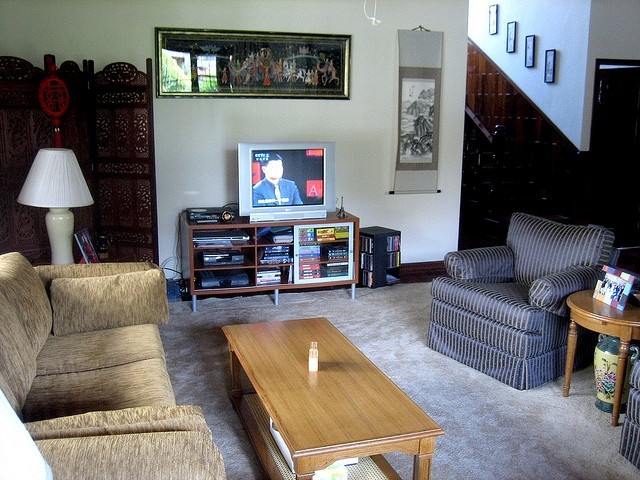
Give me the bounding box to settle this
[20,404,211,439]
[50,269,169,336]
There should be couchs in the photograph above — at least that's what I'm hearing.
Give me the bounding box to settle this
[0,252,226,480]
[426,212,616,390]
[619,360,640,470]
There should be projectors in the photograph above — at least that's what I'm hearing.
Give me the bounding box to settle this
[186,208,234,222]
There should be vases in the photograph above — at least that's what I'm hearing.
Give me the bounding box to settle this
[594,333,640,413]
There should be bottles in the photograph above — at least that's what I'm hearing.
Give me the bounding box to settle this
[95,235,110,261]
[308,341,318,372]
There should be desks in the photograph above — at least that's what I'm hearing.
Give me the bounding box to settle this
[563,289,640,427]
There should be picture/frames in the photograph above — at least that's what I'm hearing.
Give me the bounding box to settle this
[593,264,640,311]
[544,49,556,83]
[525,35,535,68]
[155,27,351,100]
[74,228,101,264]
[489,4,497,35]
[506,22,516,53]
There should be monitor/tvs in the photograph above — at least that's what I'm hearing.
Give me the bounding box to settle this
[236,141,337,222]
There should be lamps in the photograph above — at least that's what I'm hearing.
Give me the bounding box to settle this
[17,147,95,265]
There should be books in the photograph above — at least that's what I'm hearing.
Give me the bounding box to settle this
[359,235,373,253]
[321,245,348,260]
[387,235,400,251]
[257,270,283,284]
[359,253,373,272]
[300,263,321,279]
[387,252,401,269]
[359,270,374,289]
[322,263,348,277]
[258,247,291,264]
[299,228,337,243]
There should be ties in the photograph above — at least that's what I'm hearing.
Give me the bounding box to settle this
[274,184,281,205]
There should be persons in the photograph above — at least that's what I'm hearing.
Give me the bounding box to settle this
[253,152,303,206]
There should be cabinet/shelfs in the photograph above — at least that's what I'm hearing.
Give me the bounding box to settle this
[359,226,401,289]
[179,207,360,312]
